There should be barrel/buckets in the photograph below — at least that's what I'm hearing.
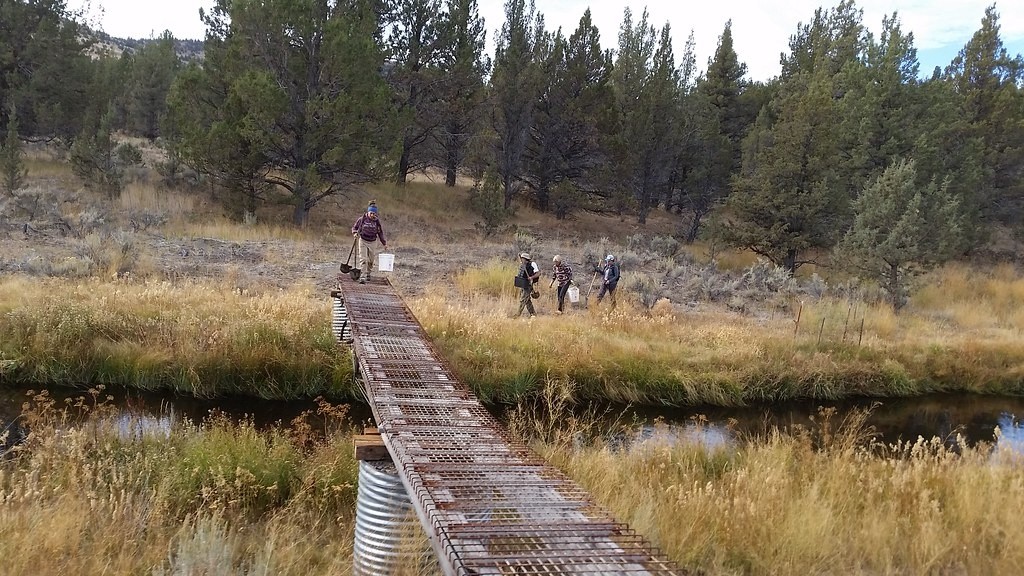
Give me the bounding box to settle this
[379,254,395,271]
[568,286,579,302]
[515,276,528,288]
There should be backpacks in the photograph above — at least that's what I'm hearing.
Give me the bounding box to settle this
[603,262,622,282]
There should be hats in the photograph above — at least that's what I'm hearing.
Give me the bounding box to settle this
[519,253,533,260]
[552,254,562,262]
[605,255,614,261]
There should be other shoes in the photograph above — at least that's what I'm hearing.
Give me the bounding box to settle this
[359,276,365,284]
[553,311,562,316]
[511,313,520,320]
[366,275,370,281]
[528,313,537,318]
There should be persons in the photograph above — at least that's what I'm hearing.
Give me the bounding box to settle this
[511,253,541,320]
[351,200,388,284]
[591,254,620,309]
[548,254,574,315]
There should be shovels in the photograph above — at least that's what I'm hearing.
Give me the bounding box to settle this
[339,212,366,281]
[518,253,540,299]
[584,260,602,307]
